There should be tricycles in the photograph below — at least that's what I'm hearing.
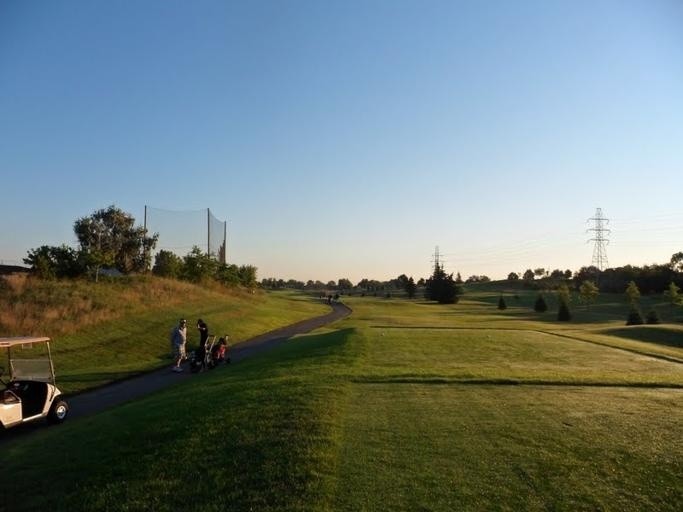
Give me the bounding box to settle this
[191,335,231,375]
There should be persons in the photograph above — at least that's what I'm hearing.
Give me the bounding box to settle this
[172,318,187,372]
[197,319,209,347]
